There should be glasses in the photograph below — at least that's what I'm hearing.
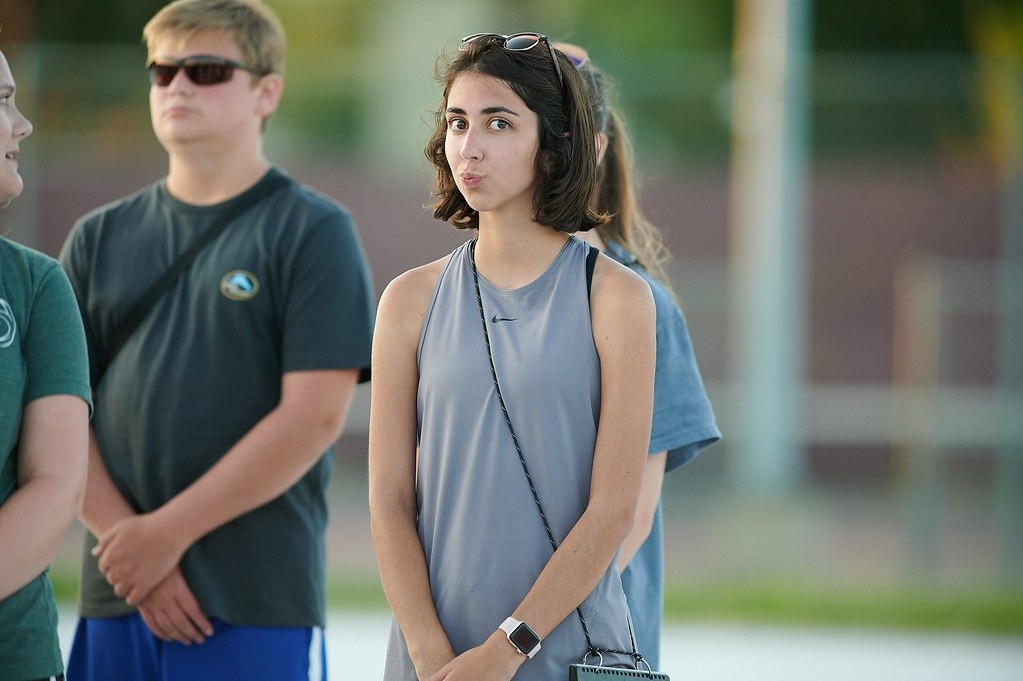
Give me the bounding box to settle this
[551,42,601,99]
[458,33,565,93]
[145,55,266,87]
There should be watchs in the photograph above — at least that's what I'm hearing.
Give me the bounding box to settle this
[497,616,542,660]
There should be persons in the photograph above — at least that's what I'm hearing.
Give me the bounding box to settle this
[368,34,657,681]
[58,1,376,681]
[1,48,94,681]
[567,108,723,681]
